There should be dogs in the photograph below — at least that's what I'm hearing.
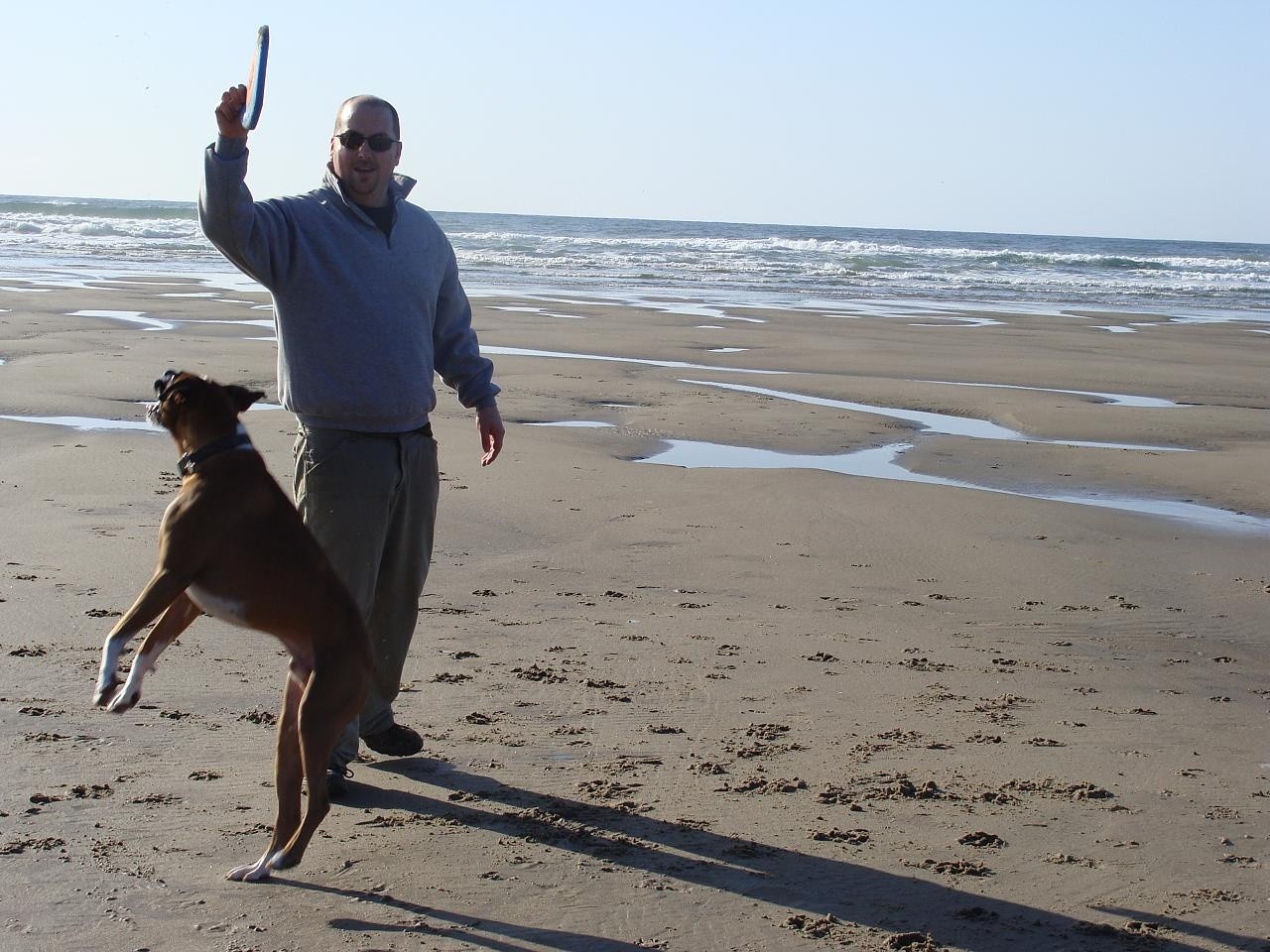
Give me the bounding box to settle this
[91,370,374,882]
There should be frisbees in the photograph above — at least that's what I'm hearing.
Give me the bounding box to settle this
[240,23,270,133]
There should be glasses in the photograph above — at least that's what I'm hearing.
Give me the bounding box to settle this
[334,131,401,152]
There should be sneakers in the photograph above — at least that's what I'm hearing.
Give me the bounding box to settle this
[302,763,356,797]
[361,724,424,757]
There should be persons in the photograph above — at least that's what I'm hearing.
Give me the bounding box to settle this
[194,80,509,801]
